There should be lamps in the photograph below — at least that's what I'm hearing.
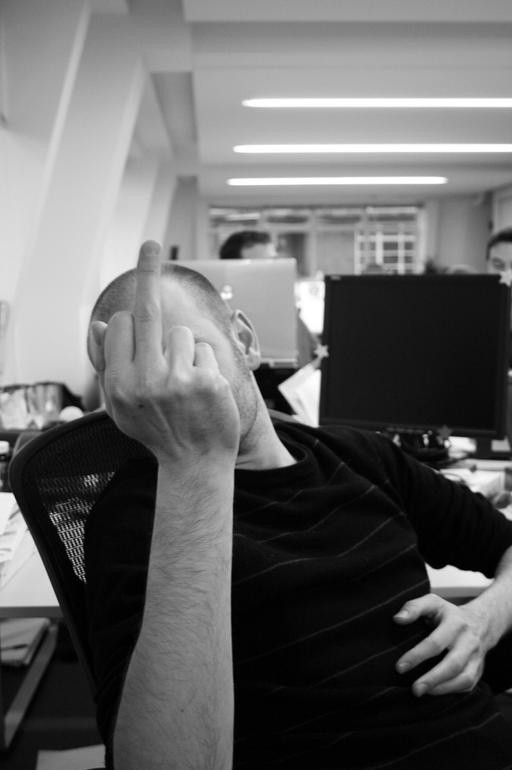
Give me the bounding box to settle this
[9,407,299,699]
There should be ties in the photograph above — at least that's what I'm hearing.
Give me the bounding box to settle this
[318,274,512,471]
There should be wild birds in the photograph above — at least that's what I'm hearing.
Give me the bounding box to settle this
[165,258,299,369]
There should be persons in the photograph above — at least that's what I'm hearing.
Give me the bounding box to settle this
[80,239,512,769]
[486,229,512,288]
[220,231,318,411]
